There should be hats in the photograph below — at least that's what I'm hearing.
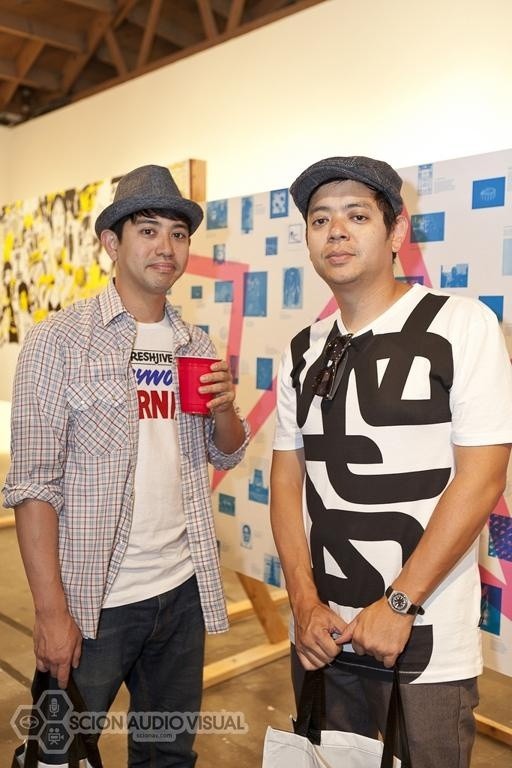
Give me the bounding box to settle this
[287,155,403,218]
[95,163,204,241]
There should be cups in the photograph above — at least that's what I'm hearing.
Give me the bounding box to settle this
[173,356,222,416]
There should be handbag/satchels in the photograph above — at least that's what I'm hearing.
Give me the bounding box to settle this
[260,647,413,768]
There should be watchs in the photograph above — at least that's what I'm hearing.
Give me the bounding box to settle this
[385,586,424,616]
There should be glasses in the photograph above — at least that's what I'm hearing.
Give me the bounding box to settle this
[312,332,354,400]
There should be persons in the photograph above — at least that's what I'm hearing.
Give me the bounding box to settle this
[269,156,512,768]
[1,165,252,768]
